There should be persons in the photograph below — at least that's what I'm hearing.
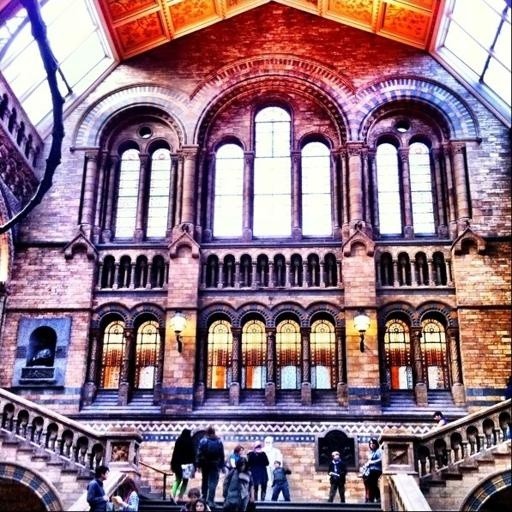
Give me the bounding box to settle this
[86,426,383,512]
[431,411,447,469]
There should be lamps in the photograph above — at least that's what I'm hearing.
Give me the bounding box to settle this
[353,308,371,353]
[170,310,187,353]
